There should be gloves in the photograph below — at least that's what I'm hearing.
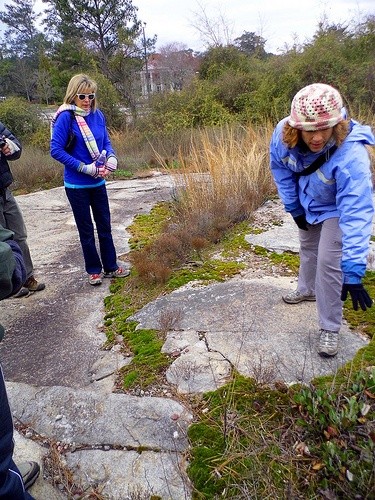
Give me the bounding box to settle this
[293,215,311,231]
[341,283,373,311]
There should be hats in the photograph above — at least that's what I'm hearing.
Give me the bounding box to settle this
[286,82,344,130]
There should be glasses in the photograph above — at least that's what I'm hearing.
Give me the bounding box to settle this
[77,94,95,100]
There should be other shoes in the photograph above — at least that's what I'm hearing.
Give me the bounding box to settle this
[16,462,40,490]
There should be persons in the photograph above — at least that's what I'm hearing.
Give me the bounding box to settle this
[0,122,45,299]
[50,74,131,284]
[269,82,375,358]
[0,220,40,500]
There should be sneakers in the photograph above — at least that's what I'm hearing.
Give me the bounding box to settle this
[318,329,339,356]
[13,286,28,297]
[282,290,316,304]
[89,274,103,285]
[104,267,130,277]
[23,276,45,291]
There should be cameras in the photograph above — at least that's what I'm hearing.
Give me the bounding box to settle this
[0,139,5,148]
